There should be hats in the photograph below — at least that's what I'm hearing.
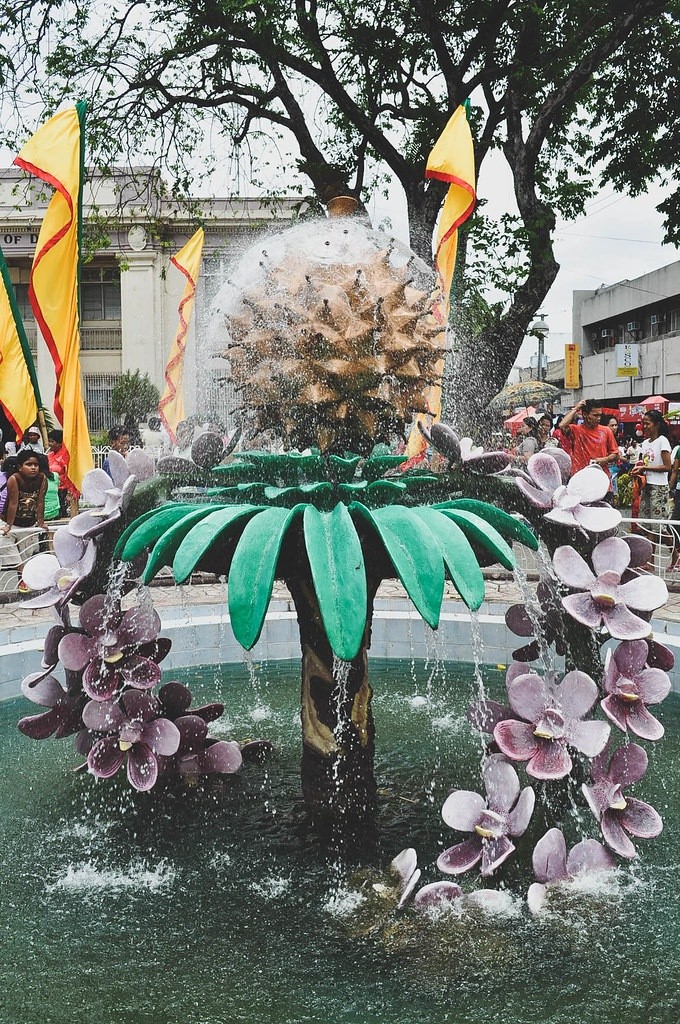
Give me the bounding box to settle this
[28,426,41,439]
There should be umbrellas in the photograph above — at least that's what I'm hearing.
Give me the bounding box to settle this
[489,381,561,417]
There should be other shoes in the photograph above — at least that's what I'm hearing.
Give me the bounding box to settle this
[18,582,29,594]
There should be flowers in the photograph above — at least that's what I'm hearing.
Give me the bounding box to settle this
[18,420,273,791]
[386,421,675,916]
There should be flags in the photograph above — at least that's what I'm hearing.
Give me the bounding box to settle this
[12,100,95,501]
[158,227,205,446]
[0,249,49,451]
[398,98,476,474]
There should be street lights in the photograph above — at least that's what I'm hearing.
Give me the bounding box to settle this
[529,315,550,382]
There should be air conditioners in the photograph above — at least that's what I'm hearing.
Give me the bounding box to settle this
[651,315,664,325]
[602,329,614,337]
[627,322,640,331]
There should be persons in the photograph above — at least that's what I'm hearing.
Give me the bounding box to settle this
[615,435,641,471]
[0,426,82,537]
[141,417,165,467]
[669,435,680,553]
[428,450,445,472]
[631,410,680,574]
[173,414,287,467]
[600,415,618,435]
[385,440,407,456]
[559,399,620,508]
[102,425,130,485]
[507,412,579,463]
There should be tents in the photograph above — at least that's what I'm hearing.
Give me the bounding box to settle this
[504,407,547,437]
